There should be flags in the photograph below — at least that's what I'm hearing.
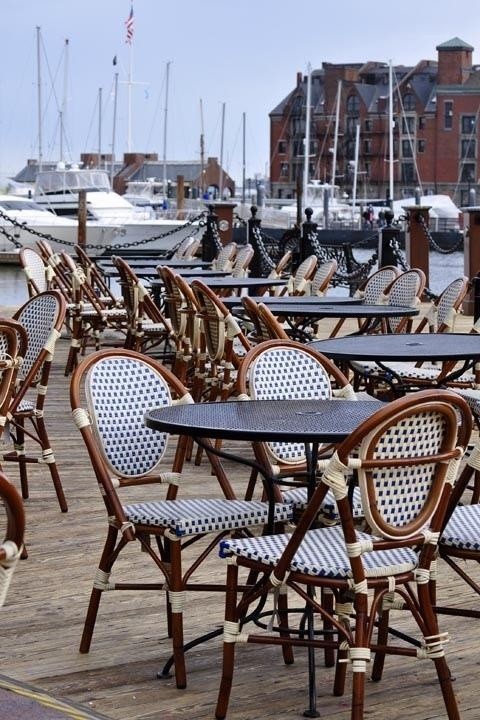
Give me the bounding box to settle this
[123,5,135,44]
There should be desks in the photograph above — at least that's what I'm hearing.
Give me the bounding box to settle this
[305,332,476,399]
[266,303,422,339]
[221,295,367,305]
[144,399,456,680]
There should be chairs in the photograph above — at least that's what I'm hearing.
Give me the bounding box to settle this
[310,258,338,299]
[11,291,68,512]
[292,255,318,295]
[435,502,480,621]
[352,318,477,390]
[345,269,427,341]
[412,275,469,368]
[69,347,296,690]
[20,235,294,404]
[237,340,371,667]
[0,317,29,440]
[0,470,25,618]
[215,389,473,720]
[339,264,404,340]
